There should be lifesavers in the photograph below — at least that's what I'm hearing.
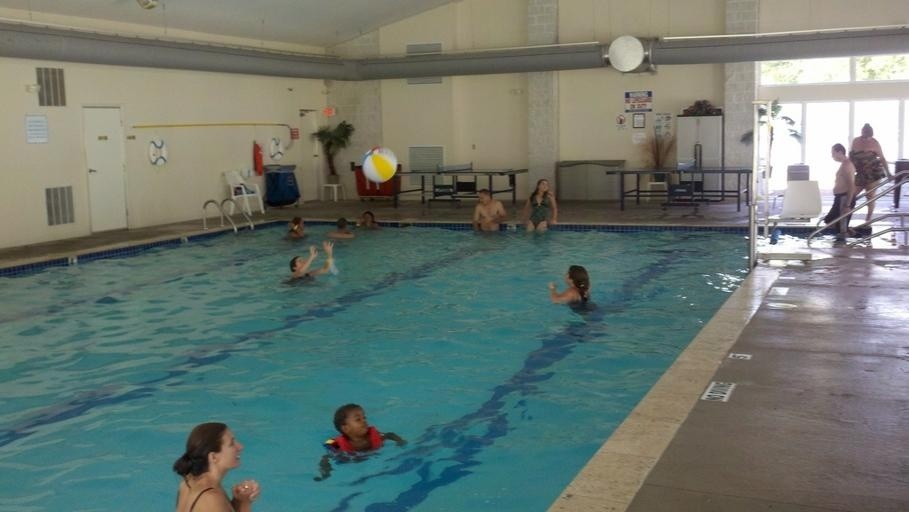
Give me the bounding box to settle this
[270,138,284,161]
[149,137,167,166]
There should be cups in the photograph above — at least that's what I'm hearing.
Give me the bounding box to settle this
[239,480,253,500]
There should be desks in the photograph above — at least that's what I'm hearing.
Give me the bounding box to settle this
[395,168,529,204]
[606,166,752,212]
[892,158,909,210]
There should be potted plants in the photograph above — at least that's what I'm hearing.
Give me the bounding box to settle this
[312,120,356,185]
[649,127,675,181]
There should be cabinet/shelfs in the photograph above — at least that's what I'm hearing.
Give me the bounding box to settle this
[675,108,723,202]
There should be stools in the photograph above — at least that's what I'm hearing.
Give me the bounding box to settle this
[322,184,346,203]
[646,182,668,201]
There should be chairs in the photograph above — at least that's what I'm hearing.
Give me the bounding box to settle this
[223,169,264,216]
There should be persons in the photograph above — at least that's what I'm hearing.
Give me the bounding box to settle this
[355,210,381,230]
[848,123,896,221]
[281,238,335,286]
[548,265,596,316]
[824,142,856,242]
[472,188,507,232]
[285,215,304,238]
[324,217,356,239]
[172,422,260,512]
[520,179,557,231]
[312,402,405,482]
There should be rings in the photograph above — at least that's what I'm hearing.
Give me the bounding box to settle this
[245,485,249,490]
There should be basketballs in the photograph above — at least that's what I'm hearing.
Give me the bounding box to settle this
[362,146,398,185]
